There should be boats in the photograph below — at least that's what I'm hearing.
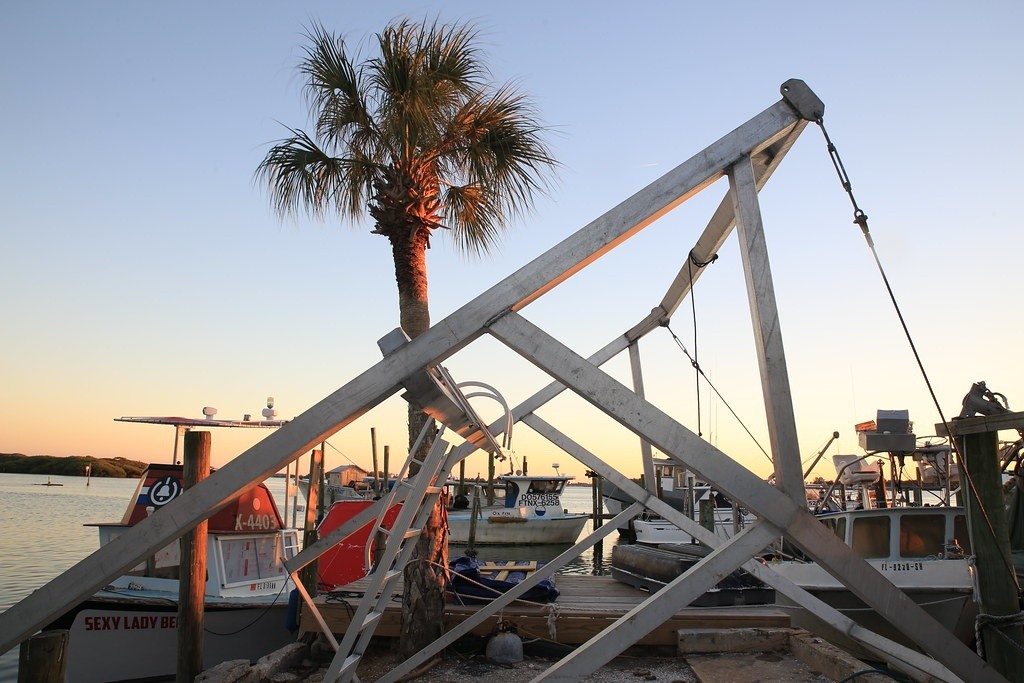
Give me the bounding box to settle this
[294,464,589,544]
[602,410,1024,662]
[84,396,303,604]
